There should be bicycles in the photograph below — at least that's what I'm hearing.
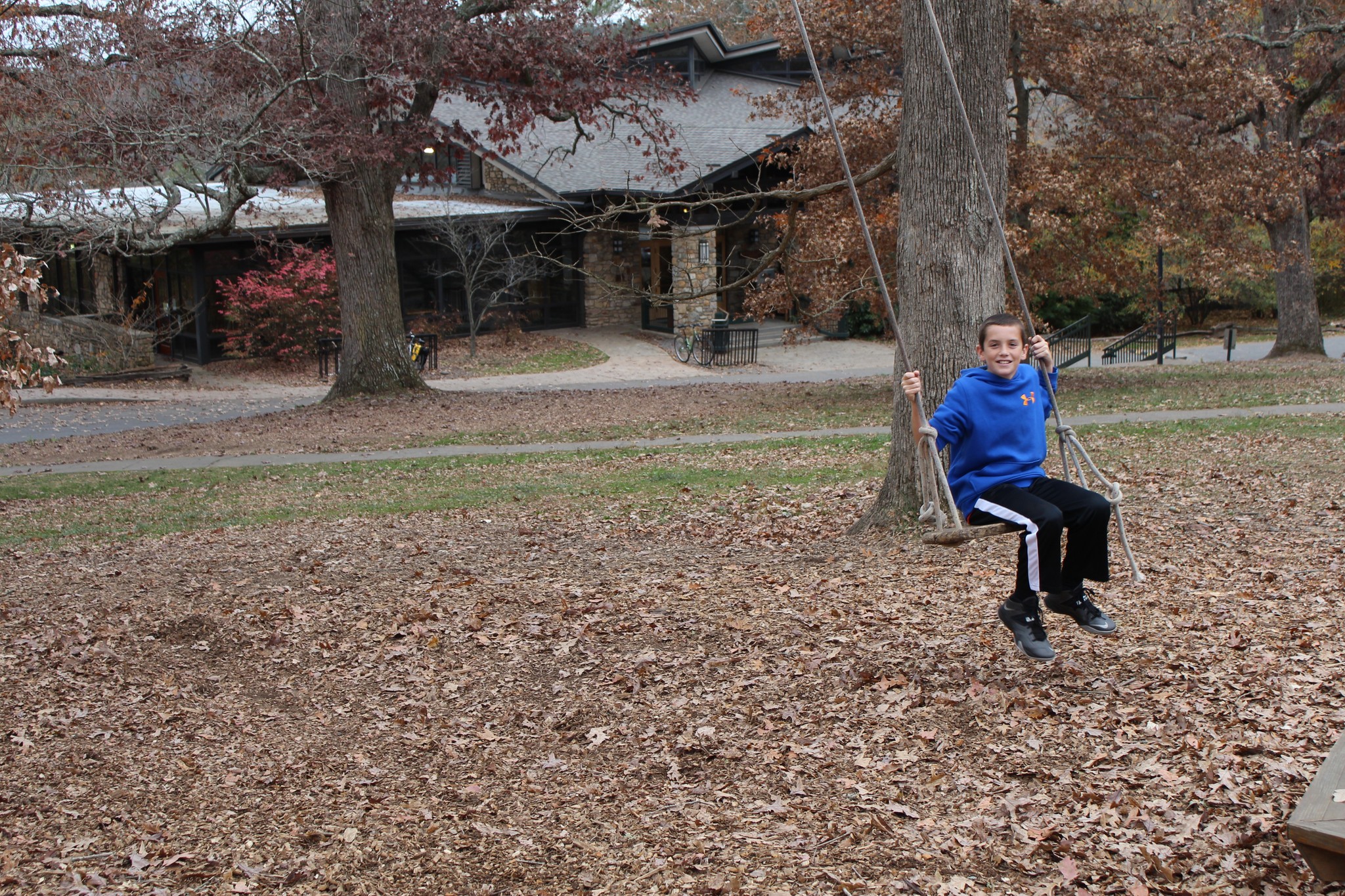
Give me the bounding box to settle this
[673,323,714,365]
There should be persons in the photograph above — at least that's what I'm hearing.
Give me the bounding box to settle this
[900,314,1116,661]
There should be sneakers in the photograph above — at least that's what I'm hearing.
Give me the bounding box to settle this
[1043,580,1116,635]
[996,593,1055,661]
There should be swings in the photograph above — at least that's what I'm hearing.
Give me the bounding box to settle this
[791,1,1114,549]
